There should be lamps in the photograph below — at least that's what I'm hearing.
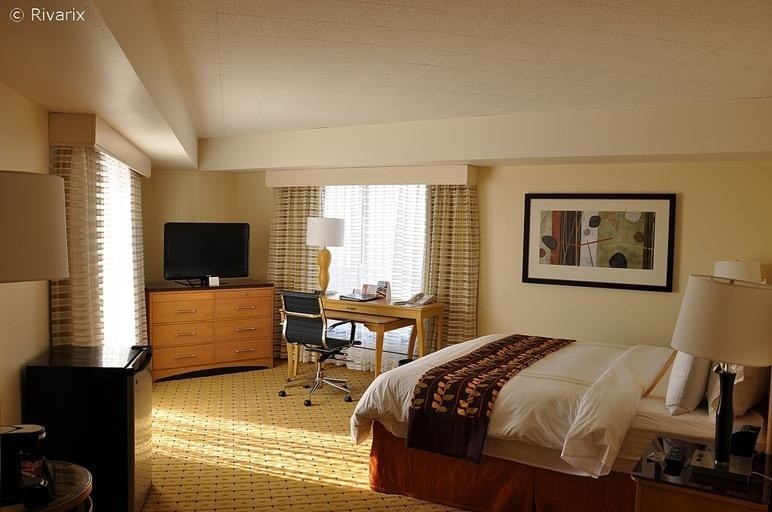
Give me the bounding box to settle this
[305,216,345,297]
[713,259,763,283]
[668,274,768,483]
[0,169,70,283]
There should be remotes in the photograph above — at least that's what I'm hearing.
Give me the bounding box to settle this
[664,446,688,467]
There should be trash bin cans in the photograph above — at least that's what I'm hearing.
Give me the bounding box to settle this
[398,359,415,366]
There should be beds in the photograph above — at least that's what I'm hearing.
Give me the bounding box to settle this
[370,333,772,512]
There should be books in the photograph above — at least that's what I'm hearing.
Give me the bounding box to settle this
[338,292,379,302]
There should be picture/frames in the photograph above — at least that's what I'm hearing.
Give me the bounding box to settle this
[521,191,678,293]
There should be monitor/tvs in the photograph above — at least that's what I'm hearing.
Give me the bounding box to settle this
[163,222,250,286]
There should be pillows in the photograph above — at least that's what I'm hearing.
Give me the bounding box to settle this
[664,351,768,422]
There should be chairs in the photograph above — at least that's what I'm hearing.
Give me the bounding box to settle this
[278,290,352,406]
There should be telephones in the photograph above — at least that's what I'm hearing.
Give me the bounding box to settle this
[408,293,434,305]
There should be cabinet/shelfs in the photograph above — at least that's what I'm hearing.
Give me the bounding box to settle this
[21,345,155,509]
[144,279,276,383]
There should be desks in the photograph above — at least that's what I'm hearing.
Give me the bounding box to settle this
[0,459,95,512]
[278,292,446,378]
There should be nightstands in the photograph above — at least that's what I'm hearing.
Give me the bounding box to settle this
[631,435,772,512]
